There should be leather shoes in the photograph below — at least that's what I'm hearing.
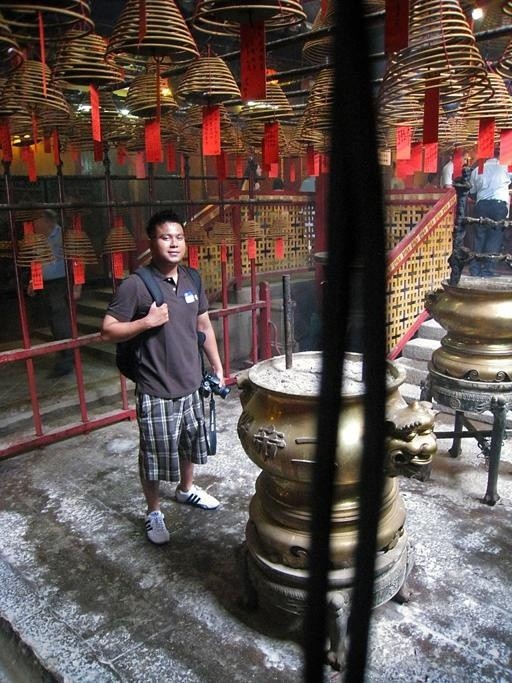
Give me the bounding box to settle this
[470,270,501,277]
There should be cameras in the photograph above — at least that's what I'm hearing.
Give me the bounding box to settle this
[202,372,231,400]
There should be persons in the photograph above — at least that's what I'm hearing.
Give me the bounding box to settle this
[26,206,83,379]
[238,160,263,200]
[101,207,226,546]
[467,144,511,276]
[440,147,469,189]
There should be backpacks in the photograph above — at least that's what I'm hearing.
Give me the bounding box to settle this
[116,263,202,385]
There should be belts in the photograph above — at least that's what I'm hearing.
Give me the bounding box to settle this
[479,199,507,206]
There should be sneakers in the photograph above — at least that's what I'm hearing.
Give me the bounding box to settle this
[175,483,221,510]
[143,509,171,545]
[48,365,72,378]
